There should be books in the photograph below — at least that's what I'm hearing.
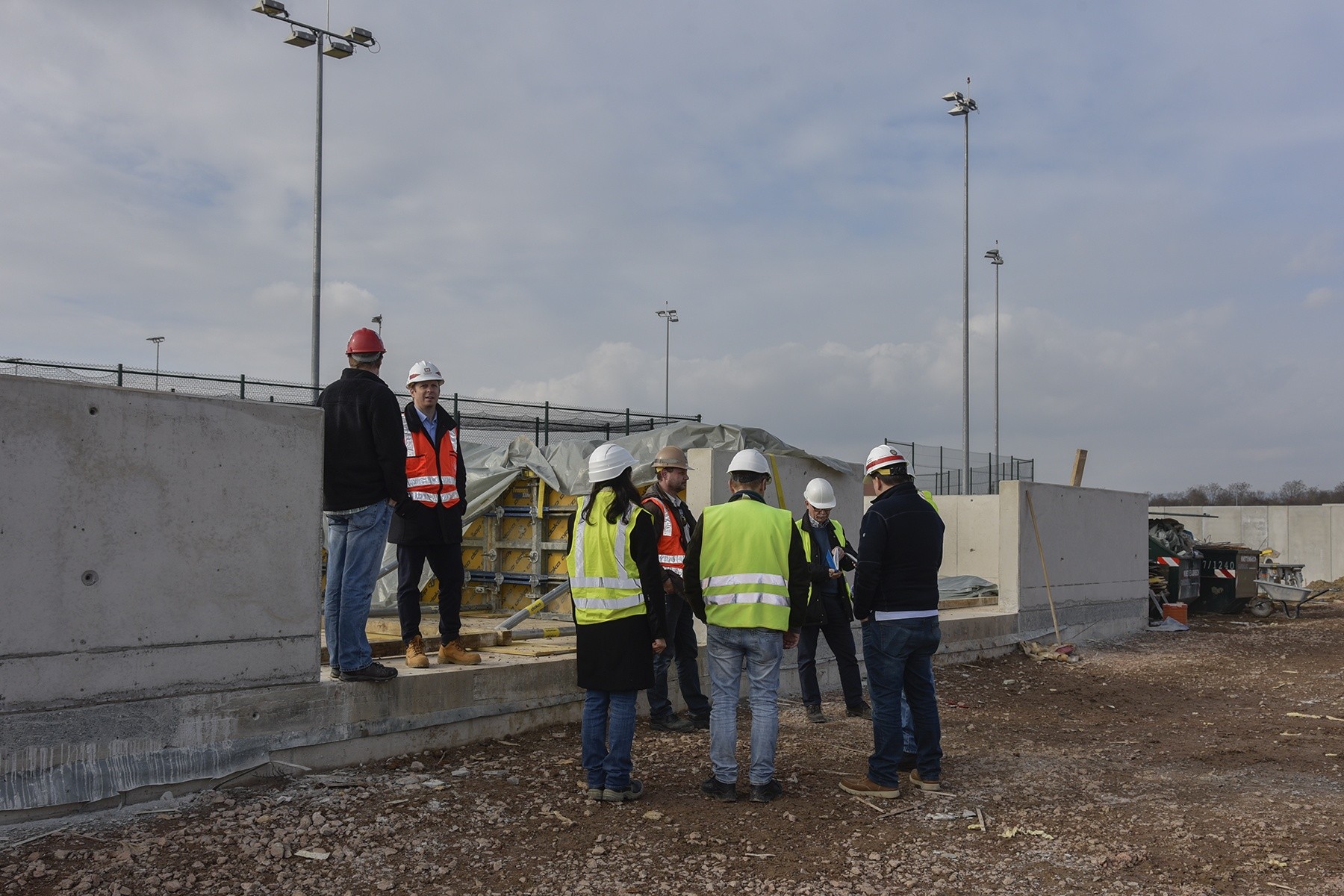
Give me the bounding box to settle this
[826,548,839,572]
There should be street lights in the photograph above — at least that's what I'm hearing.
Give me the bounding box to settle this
[942,89,978,501]
[655,309,679,427]
[251,0,382,407]
[145,336,166,390]
[984,248,1004,494]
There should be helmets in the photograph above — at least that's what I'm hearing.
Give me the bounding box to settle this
[725,449,772,484]
[406,360,444,388]
[345,327,386,354]
[588,443,639,483]
[863,444,910,485]
[804,478,836,508]
[651,445,694,470]
[906,459,917,479]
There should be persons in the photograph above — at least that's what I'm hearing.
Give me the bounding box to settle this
[565,444,666,800]
[899,463,942,772]
[640,445,712,731]
[837,444,945,800]
[316,328,407,681]
[794,477,873,723]
[388,360,481,667]
[682,449,809,803]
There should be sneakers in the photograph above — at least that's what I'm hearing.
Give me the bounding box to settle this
[330,667,341,678]
[806,704,825,722]
[588,786,604,799]
[650,713,694,732]
[437,639,481,665]
[406,635,429,667]
[910,768,939,790]
[691,707,712,728]
[838,776,900,798]
[846,701,873,720]
[339,659,398,682]
[702,773,738,802]
[750,780,781,802]
[602,778,644,801]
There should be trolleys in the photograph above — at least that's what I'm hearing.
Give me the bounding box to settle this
[1244,579,1341,620]
